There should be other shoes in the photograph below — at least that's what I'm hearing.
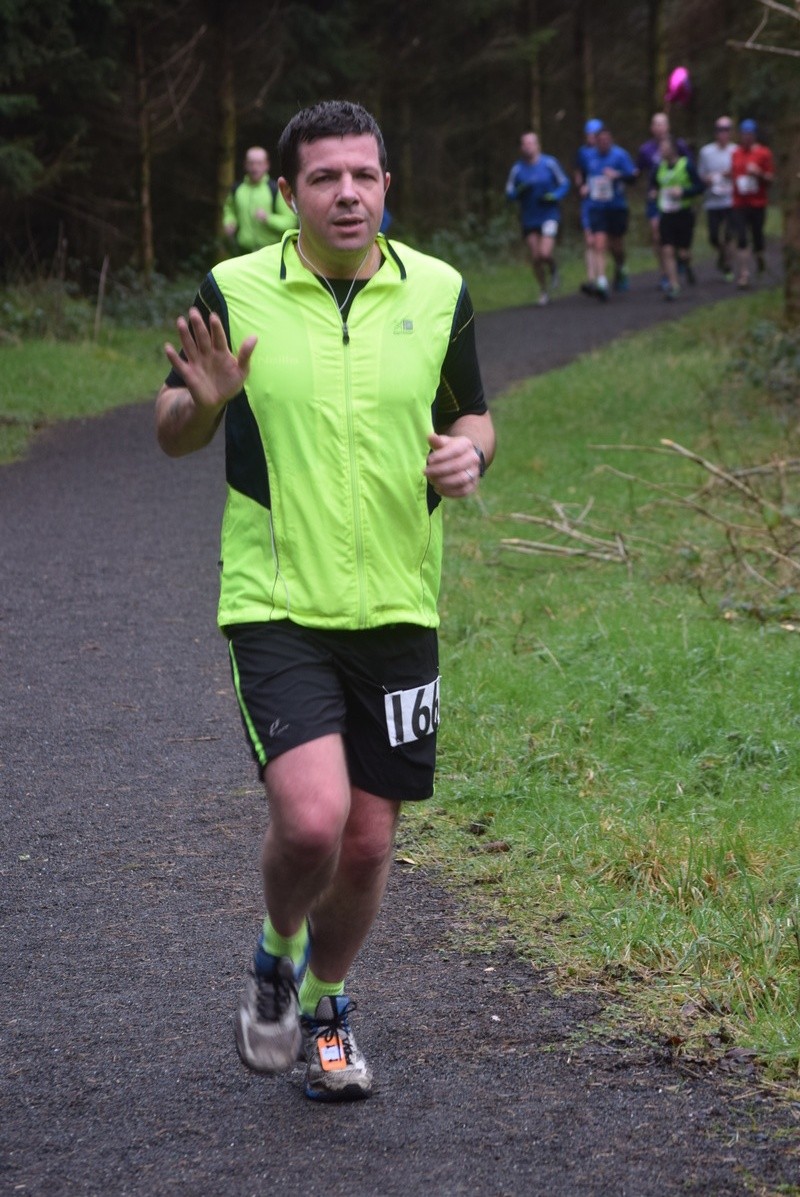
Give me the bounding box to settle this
[667,284,682,299]
[685,268,695,282]
[595,286,610,301]
[658,277,669,290]
[737,274,751,287]
[756,259,767,272]
[582,281,597,295]
[551,271,559,288]
[615,274,627,292]
[537,294,550,306]
[724,270,735,283]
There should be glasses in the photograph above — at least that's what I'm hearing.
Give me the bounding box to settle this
[716,125,730,132]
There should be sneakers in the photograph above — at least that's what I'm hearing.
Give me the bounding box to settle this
[297,995,374,1102]
[234,915,312,1075]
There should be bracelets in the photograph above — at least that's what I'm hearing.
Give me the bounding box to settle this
[474,445,485,477]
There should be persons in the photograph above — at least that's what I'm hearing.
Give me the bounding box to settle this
[504,113,777,307]
[154,100,498,1105]
[222,146,298,257]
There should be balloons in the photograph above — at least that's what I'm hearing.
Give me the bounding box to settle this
[663,67,691,102]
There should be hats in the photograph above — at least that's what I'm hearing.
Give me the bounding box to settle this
[740,119,757,134]
[585,119,603,133]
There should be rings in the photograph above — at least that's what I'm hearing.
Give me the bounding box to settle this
[465,469,474,483]
